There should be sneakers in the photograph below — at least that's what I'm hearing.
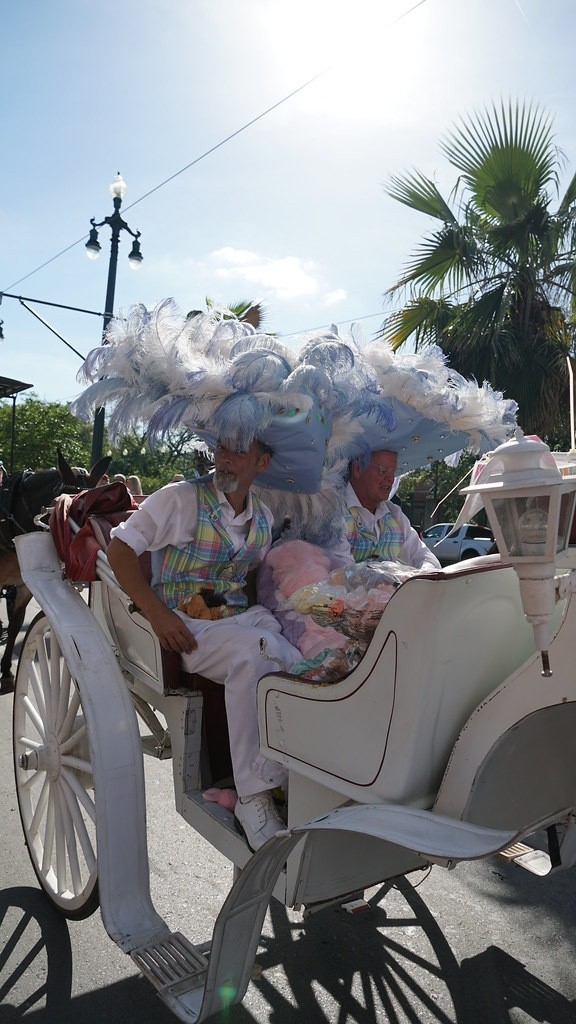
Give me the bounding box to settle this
[233,791,289,867]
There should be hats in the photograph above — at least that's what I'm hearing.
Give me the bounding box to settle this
[114,474,126,482]
[171,474,186,482]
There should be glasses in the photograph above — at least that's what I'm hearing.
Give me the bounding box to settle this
[369,463,398,476]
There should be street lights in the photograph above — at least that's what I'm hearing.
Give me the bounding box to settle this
[83,172,144,472]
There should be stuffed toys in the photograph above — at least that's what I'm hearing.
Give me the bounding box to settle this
[174,591,231,620]
[269,540,419,675]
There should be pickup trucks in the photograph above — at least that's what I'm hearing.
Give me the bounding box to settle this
[419,523,497,562]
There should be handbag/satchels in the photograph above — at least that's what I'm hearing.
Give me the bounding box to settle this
[49,482,139,580]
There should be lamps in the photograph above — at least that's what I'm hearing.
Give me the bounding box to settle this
[459,428,576,677]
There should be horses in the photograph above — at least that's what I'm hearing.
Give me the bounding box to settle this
[0,448,112,695]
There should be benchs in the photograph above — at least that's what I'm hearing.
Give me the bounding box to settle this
[89,509,235,792]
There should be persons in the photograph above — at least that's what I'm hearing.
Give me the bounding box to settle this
[323,449,444,582]
[103,473,143,494]
[106,433,322,855]
